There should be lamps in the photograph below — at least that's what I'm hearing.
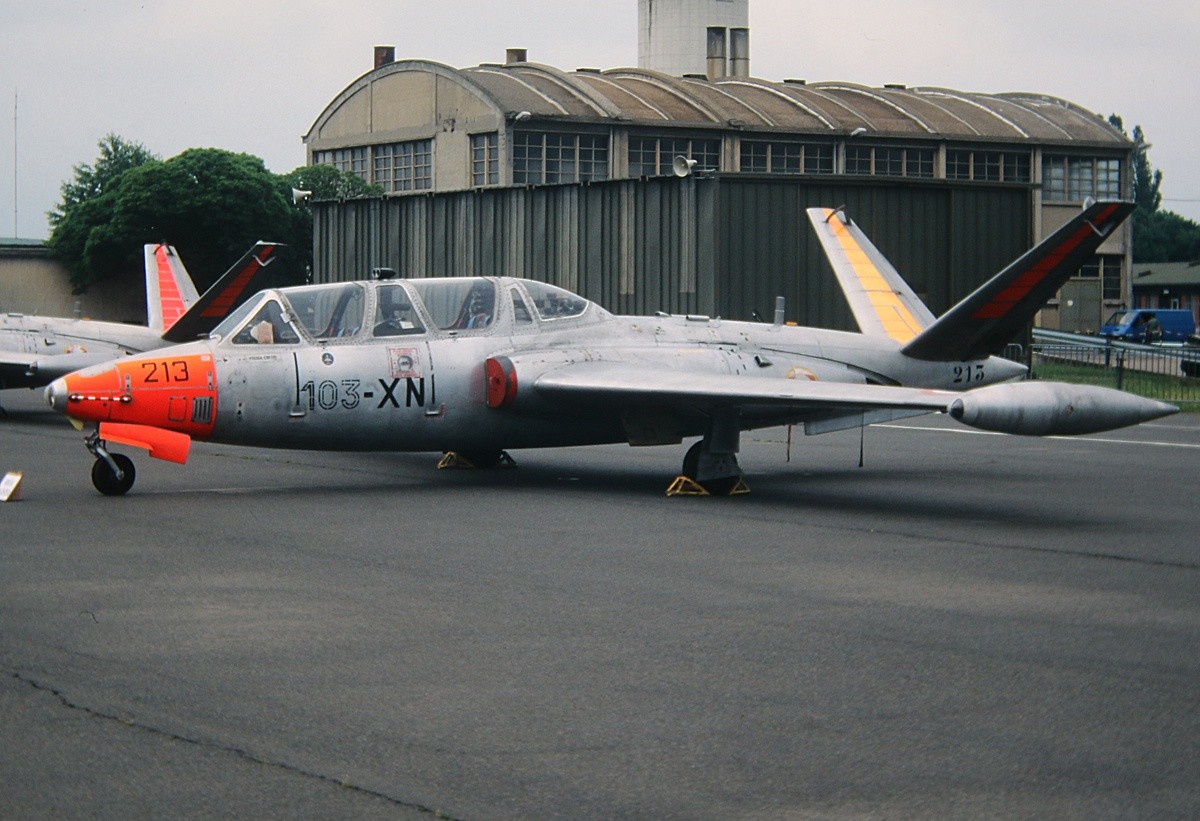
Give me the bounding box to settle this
[507,110,531,137]
[843,126,867,145]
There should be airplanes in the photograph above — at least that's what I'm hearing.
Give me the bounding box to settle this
[44,197,1180,509]
[0,241,288,420]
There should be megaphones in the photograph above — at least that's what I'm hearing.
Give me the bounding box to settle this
[291,187,312,205]
[673,155,697,177]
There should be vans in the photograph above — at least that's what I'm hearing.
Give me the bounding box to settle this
[1097,309,1195,345]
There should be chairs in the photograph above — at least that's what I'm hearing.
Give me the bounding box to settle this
[466,312,490,330]
